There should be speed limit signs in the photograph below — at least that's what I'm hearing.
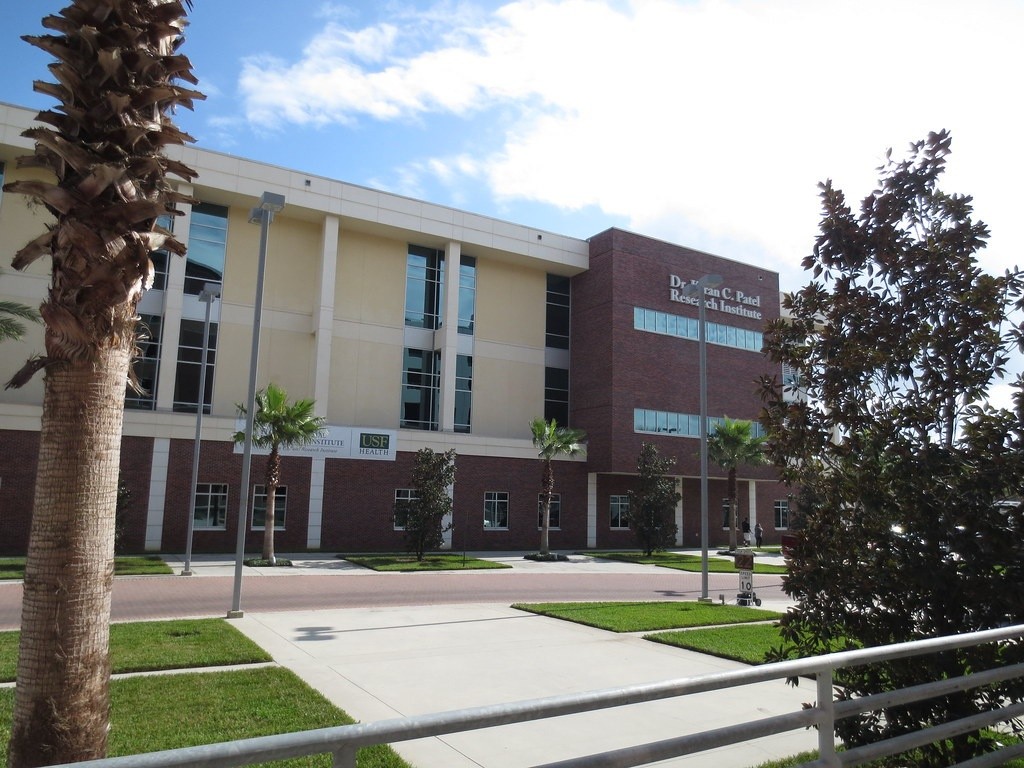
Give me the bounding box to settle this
[737,570,753,593]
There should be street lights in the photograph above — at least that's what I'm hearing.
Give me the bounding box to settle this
[179,283,223,570]
[225,193,285,616]
[682,275,721,604]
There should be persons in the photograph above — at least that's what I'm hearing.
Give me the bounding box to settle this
[741,517,753,547]
[755,523,764,549]
